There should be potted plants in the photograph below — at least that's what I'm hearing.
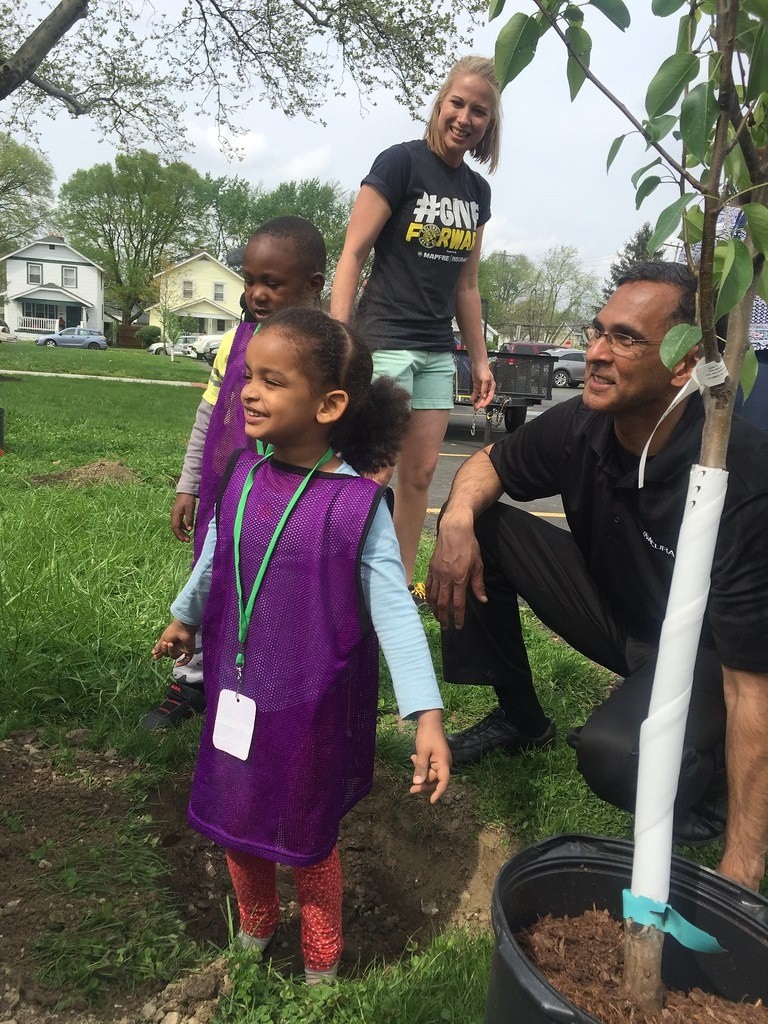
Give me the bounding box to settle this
[480,0,768,1024]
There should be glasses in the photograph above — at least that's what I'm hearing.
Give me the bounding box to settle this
[581,324,663,358]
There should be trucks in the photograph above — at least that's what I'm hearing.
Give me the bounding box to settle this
[453,348,559,430]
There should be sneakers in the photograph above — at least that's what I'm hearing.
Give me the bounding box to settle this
[304,962,341,988]
[630,768,735,848]
[234,930,277,954]
[412,706,558,776]
[407,582,428,609]
[138,680,208,735]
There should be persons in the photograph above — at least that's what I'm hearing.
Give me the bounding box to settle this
[327,60,502,590]
[424,259,768,895]
[151,215,453,987]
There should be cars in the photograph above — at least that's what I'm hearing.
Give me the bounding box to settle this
[34,327,107,351]
[530,348,589,388]
[145,327,237,368]
[0,319,11,343]
[453,337,466,351]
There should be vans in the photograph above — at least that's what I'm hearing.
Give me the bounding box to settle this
[494,343,571,385]
